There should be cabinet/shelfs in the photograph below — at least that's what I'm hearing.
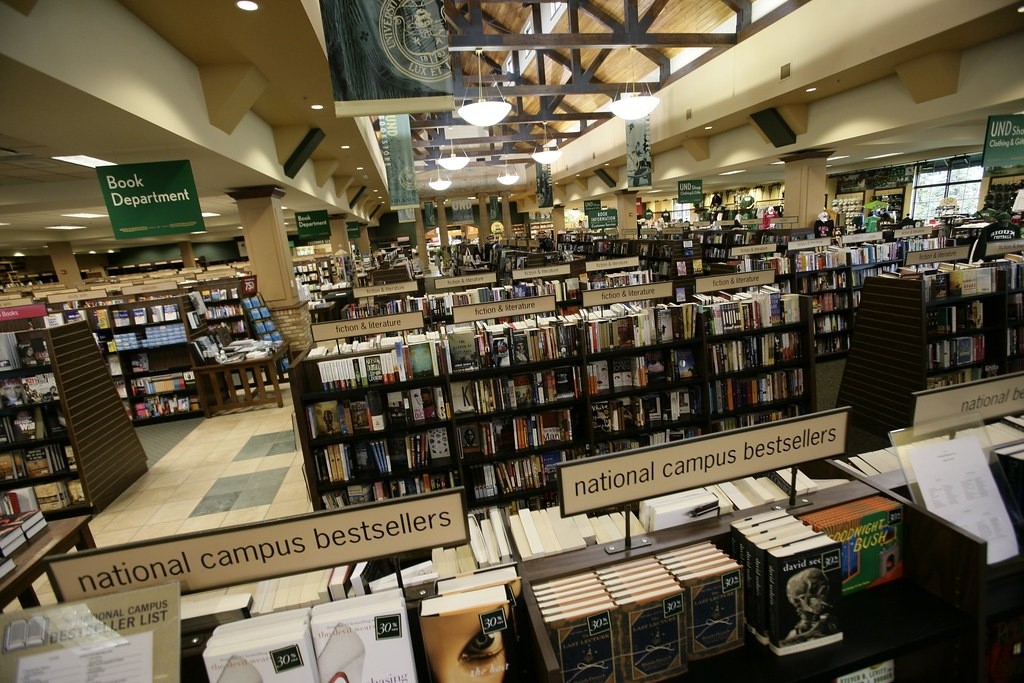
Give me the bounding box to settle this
[0,187,1023,683]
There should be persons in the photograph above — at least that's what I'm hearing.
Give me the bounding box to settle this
[812,212,834,237]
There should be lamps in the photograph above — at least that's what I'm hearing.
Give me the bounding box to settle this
[610,47,660,121]
[530,123,561,165]
[430,166,451,190]
[458,51,512,127]
[498,154,519,185]
[438,128,471,171]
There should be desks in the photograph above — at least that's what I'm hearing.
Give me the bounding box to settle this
[310,301,339,323]
[0,515,96,614]
[190,340,294,418]
[324,290,357,316]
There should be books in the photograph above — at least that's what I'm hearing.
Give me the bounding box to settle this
[0,413,1024,683]
[1,232,1023,574]
